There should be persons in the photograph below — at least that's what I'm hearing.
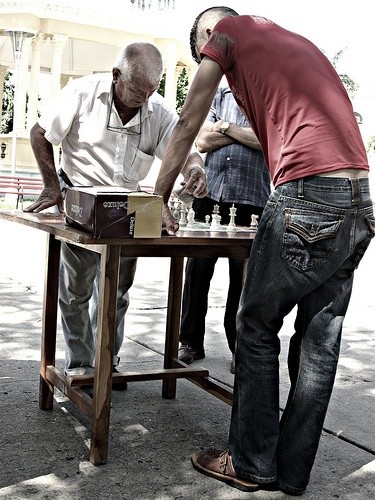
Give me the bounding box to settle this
[153,6,375,495]
[23,43,209,397]
[178,84,271,374]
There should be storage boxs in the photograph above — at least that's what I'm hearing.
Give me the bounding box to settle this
[63,186,162,238]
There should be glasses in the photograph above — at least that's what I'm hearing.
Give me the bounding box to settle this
[106,96,141,135]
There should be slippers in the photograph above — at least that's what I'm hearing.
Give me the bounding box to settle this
[192,446,260,491]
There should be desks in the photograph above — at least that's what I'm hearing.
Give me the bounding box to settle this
[0,210,300,470]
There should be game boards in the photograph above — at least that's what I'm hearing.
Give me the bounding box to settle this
[173,218,259,234]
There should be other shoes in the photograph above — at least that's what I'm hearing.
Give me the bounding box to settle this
[112,369,127,391]
[80,385,93,397]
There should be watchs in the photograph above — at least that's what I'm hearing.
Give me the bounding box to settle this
[220,122,232,133]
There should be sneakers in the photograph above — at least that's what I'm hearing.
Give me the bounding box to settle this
[178,344,205,360]
[230,353,236,374]
